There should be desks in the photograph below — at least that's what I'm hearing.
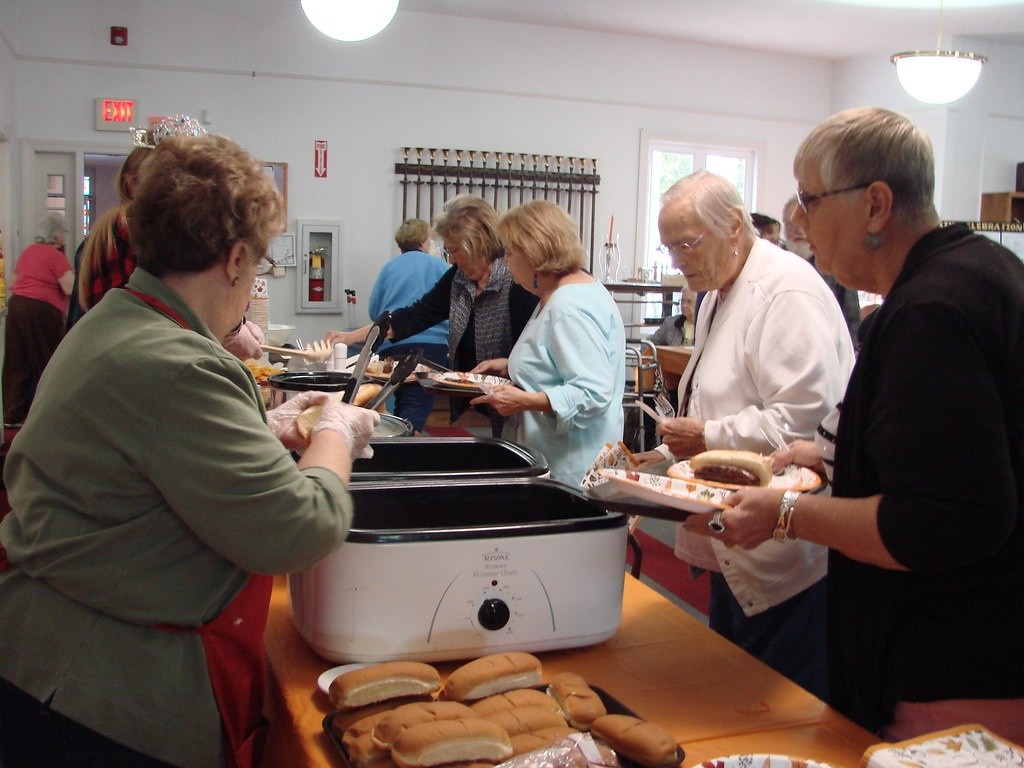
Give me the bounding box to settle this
[635,345,693,393]
[266,571,885,767]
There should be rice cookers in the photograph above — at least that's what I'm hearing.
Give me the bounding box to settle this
[289,436,550,483]
[372,413,415,438]
[288,477,629,665]
[267,371,352,409]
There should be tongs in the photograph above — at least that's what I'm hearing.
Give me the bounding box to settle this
[340,310,424,411]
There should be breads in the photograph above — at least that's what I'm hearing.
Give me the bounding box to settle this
[296,383,387,439]
[328,652,678,768]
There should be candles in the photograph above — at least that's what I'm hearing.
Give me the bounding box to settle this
[609,214,614,244]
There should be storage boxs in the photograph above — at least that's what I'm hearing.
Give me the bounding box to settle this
[662,274,687,286]
[253,279,268,298]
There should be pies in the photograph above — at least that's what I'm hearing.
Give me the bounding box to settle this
[306,340,332,354]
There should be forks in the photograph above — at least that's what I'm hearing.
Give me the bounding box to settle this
[653,395,675,419]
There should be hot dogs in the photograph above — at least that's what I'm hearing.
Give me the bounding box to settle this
[690,450,772,488]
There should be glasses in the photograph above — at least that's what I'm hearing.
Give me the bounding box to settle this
[442,246,462,257]
[656,217,720,254]
[227,238,276,276]
[796,182,894,213]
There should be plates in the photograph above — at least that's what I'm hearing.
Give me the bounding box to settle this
[316,666,371,695]
[598,468,733,515]
[666,456,822,491]
[434,371,514,387]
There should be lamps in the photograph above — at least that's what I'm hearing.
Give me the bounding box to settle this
[891,51,988,105]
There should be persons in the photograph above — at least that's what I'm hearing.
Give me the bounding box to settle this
[0,114,382,768]
[65,115,266,362]
[370,219,454,432]
[749,193,860,353]
[469,199,626,495]
[648,285,697,417]
[682,104,1024,756]
[626,171,856,714]
[324,193,539,443]
[2,208,75,429]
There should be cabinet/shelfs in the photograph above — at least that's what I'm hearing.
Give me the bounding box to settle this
[602,282,683,344]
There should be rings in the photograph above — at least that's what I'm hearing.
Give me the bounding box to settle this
[708,510,725,533]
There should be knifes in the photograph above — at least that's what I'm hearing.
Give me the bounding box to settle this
[635,400,661,424]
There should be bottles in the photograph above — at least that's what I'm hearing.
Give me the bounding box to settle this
[599,232,621,283]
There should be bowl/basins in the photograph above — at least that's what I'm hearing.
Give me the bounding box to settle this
[690,753,833,768]
[268,324,296,347]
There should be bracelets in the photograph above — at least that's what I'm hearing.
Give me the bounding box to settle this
[774,488,801,544]
[701,429,706,446]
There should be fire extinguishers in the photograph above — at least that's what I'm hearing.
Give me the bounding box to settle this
[309,248,327,302]
